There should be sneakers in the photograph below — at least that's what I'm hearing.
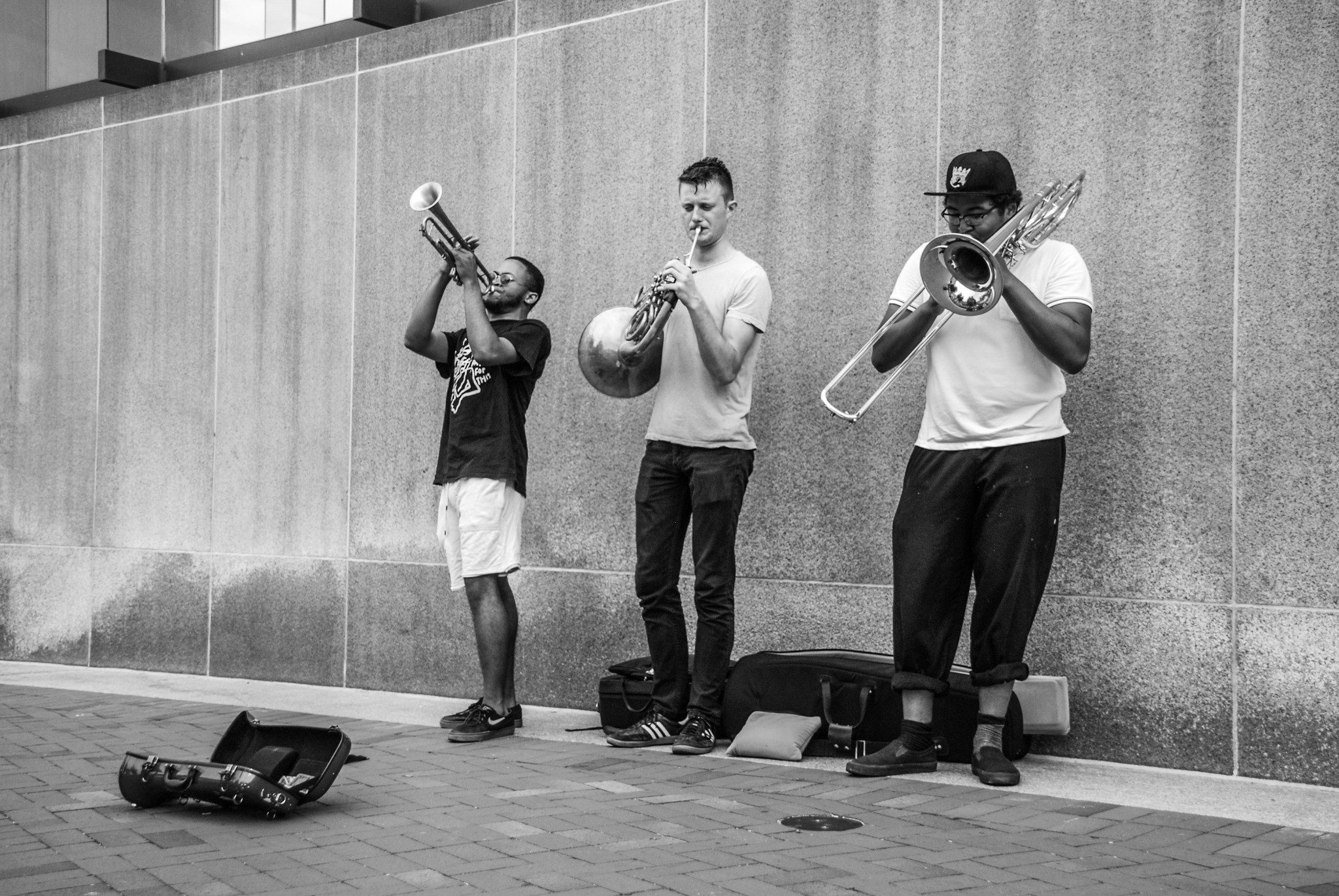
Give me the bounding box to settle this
[673,716,714,754]
[607,708,689,747]
[439,696,522,730]
[448,705,515,741]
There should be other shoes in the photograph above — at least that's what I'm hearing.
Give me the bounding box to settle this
[846,739,937,776]
[971,745,1020,785]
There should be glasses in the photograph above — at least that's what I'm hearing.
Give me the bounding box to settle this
[487,272,534,292]
[941,202,1005,227]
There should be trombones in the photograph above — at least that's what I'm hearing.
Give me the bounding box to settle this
[822,172,1094,423]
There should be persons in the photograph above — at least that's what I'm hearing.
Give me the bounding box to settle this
[405,233,552,744]
[846,148,1093,786]
[606,158,772,756]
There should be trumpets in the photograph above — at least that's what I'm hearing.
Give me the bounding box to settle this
[407,179,495,301]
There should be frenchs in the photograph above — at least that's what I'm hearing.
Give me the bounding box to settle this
[578,234,700,403]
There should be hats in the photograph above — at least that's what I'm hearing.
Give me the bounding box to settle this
[924,150,1016,197]
